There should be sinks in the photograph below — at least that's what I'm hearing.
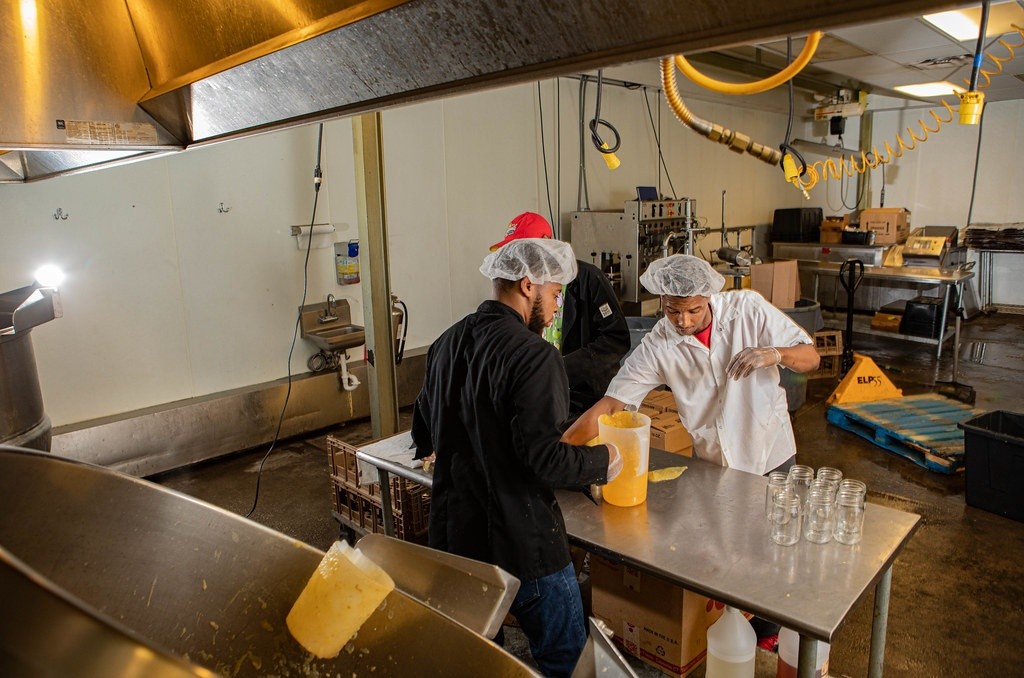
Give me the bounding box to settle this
[314,325,365,338]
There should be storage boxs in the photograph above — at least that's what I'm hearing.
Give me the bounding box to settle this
[739,257,843,381]
[586,550,756,678]
[955,408,1024,525]
[817,213,852,244]
[634,387,694,458]
[326,430,443,541]
[860,207,958,270]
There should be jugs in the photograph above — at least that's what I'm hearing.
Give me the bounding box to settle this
[285,539,395,659]
[598,411,651,507]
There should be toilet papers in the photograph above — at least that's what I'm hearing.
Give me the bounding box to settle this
[298,225,334,250]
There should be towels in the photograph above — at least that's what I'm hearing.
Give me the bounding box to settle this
[356,429,436,485]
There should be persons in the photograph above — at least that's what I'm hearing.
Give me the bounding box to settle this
[490,212,631,436]
[411,238,623,678]
[557,254,820,654]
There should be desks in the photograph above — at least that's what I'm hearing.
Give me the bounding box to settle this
[801,262,974,380]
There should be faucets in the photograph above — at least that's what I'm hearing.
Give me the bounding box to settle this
[326,293,336,316]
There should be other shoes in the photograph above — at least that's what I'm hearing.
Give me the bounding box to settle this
[749,616,781,654]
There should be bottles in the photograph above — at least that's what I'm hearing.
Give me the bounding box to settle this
[705,605,757,678]
[776,627,831,678]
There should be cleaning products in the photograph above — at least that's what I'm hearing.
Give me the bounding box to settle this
[335,242,362,286]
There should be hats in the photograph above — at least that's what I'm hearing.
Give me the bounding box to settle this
[489,212,552,251]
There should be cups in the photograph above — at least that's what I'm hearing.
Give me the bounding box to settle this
[765,465,866,546]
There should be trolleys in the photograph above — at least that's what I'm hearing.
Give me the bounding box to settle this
[825,260,995,475]
